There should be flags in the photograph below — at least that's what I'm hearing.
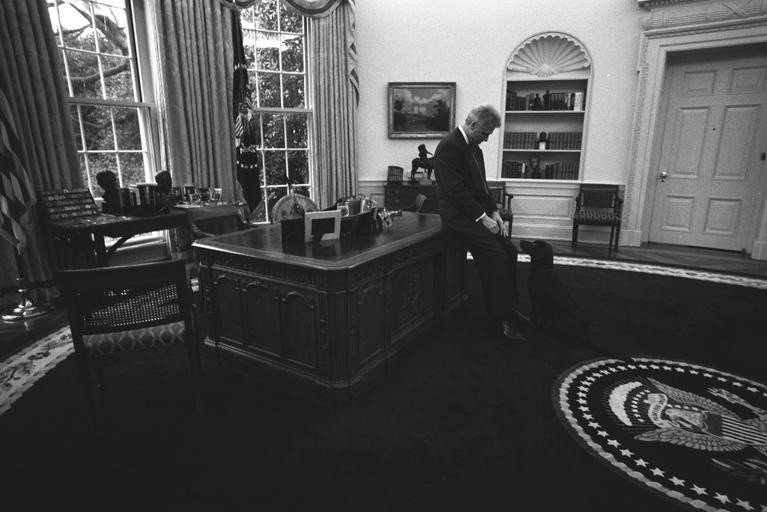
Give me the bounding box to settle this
[0,71,40,256]
[228,4,262,212]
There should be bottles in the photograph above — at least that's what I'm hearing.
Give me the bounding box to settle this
[537,131,548,151]
[534,93,542,111]
[543,90,552,110]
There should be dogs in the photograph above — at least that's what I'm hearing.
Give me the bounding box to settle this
[520,239,591,334]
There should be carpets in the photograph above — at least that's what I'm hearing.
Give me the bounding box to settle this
[1,251,764,503]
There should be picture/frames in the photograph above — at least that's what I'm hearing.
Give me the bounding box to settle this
[310,210,376,245]
[385,81,456,139]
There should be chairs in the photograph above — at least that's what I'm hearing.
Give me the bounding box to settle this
[53,262,205,418]
[570,181,624,251]
[272,192,316,222]
[402,193,427,213]
[482,182,513,240]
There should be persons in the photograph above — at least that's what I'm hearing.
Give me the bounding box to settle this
[431,103,532,344]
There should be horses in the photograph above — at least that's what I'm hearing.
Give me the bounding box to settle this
[410,156,435,182]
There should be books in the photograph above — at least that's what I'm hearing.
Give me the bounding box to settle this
[500,90,585,180]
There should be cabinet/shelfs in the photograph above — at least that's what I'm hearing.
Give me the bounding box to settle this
[497,80,593,246]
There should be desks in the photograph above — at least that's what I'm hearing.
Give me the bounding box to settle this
[191,204,491,407]
[385,174,511,243]
[35,190,241,268]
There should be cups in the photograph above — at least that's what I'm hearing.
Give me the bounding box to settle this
[544,163,555,179]
[334,203,349,217]
[346,197,361,216]
[213,188,223,200]
[573,92,585,112]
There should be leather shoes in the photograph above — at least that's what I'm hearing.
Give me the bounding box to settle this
[499,321,527,341]
[510,306,531,326]
